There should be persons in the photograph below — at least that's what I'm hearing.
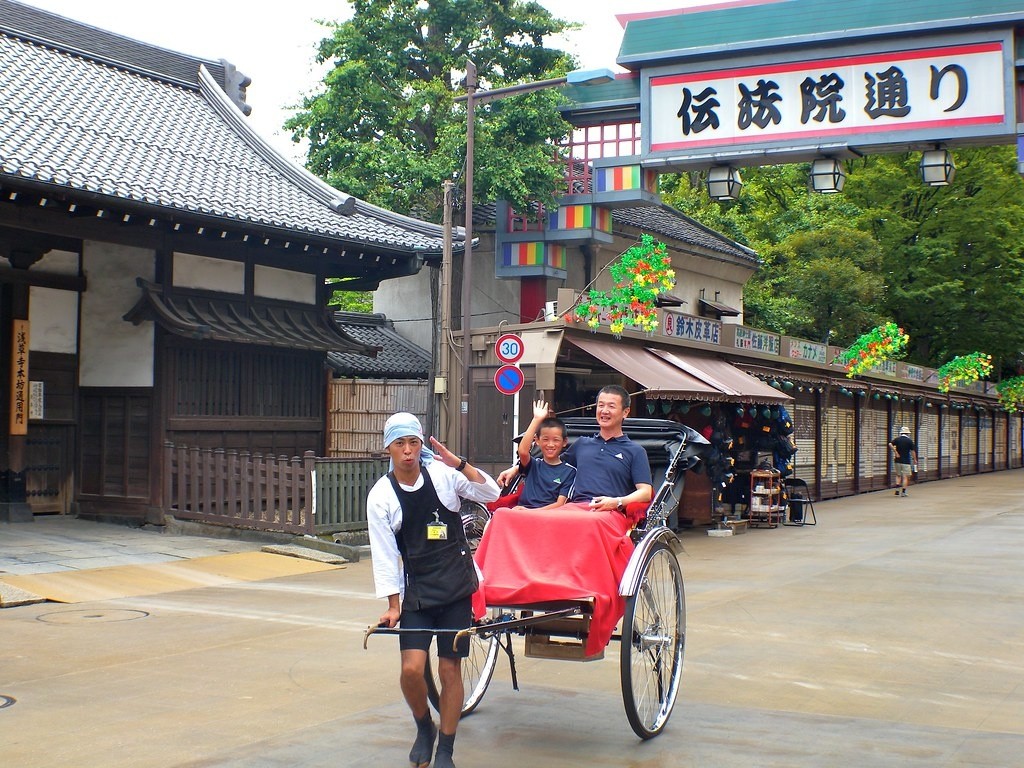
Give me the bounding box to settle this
[497,384,652,511]
[440,531,445,538]
[888,427,917,497]
[366,412,500,768]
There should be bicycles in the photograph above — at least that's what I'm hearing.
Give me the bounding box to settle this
[459,496,493,550]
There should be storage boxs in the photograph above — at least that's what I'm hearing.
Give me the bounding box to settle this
[707,530,733,537]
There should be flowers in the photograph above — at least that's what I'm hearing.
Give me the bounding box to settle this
[835,320,909,377]
[550,233,675,332]
[995,376,1024,414]
[937,351,993,392]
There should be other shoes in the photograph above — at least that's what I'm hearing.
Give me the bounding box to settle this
[894,490,899,496]
[902,489,906,496]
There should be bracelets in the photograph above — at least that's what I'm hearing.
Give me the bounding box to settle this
[456,456,467,471]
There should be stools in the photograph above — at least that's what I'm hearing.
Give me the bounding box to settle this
[715,520,748,535]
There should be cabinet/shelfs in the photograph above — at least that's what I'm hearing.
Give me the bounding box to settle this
[749,471,781,528]
[710,449,787,529]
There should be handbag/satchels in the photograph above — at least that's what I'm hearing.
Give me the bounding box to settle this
[769,407,798,507]
[700,416,738,504]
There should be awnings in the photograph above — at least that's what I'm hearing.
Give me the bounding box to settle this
[554,336,795,415]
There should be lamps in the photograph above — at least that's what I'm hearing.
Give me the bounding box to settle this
[660,401,674,414]
[772,406,780,419]
[769,379,781,389]
[645,400,657,415]
[749,405,757,418]
[918,146,957,186]
[780,380,794,391]
[761,405,770,419]
[808,158,845,194]
[693,402,711,416]
[704,166,742,202]
[734,404,745,417]
[678,401,692,414]
[799,385,1006,412]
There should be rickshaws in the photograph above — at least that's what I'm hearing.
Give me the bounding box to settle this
[364,418,710,739]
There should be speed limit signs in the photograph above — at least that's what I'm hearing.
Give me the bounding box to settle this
[495,334,525,363]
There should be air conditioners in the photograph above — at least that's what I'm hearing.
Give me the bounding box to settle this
[545,300,558,322]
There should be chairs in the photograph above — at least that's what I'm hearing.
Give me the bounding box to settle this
[782,478,817,527]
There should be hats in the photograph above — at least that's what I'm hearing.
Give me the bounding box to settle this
[899,427,912,434]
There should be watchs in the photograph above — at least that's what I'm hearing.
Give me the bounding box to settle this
[615,496,623,511]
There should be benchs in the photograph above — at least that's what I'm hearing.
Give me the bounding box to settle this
[510,439,677,523]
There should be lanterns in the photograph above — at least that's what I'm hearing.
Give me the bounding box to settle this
[809,158,845,193]
[708,166,742,200]
[920,150,956,186]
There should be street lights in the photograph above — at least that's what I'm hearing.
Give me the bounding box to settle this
[453,59,615,459]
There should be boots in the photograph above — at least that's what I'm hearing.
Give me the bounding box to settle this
[434,729,456,768]
[409,709,437,768]
